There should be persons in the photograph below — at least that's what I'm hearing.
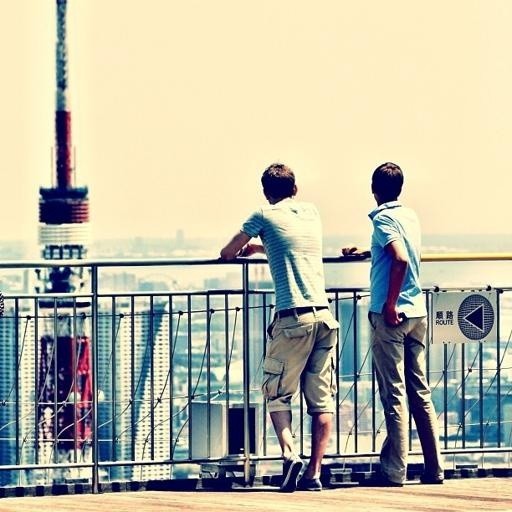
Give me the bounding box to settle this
[220,164,340,491]
[343,163,445,487]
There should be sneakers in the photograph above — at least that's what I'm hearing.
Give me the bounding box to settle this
[279,452,323,492]
[420,476,444,484]
[359,471,403,487]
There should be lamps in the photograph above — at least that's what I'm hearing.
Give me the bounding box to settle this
[190,400,261,472]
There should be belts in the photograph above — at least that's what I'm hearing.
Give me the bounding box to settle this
[274,306,328,318]
